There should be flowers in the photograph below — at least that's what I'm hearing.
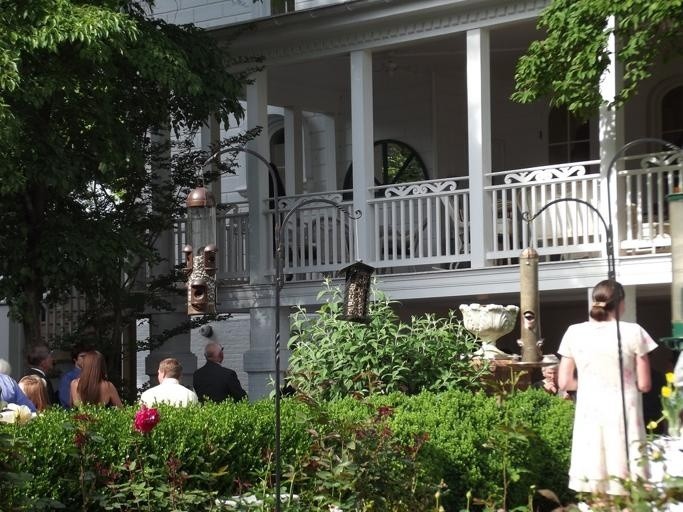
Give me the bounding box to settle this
[646,372,683,438]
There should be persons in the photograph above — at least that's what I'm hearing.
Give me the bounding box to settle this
[558,281,659,512]
[0,344,54,424]
[532,354,559,395]
[58,345,122,408]
[193,342,247,405]
[140,358,198,410]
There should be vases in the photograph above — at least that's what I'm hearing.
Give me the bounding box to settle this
[663,438,683,477]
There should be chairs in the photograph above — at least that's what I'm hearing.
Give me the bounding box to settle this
[308,216,348,278]
[516,180,601,261]
[379,217,427,273]
[440,196,512,270]
